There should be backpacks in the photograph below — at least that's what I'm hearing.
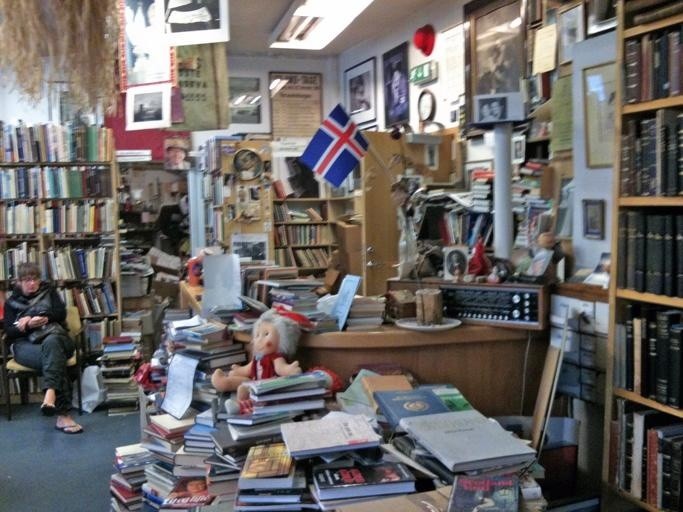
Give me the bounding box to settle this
[5,288,66,344]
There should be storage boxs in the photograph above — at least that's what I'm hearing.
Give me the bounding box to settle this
[148,265,180,299]
[117,269,154,299]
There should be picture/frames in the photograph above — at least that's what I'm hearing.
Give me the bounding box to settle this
[443,244,471,282]
[580,62,616,172]
[460,0,532,122]
[381,38,409,129]
[583,0,617,39]
[342,53,377,126]
[356,124,379,133]
[122,81,173,132]
[581,198,604,239]
[553,0,589,70]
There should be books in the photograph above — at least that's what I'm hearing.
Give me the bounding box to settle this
[2,2,683,512]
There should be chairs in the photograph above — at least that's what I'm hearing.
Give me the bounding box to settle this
[494,345,565,465]
[1,305,87,417]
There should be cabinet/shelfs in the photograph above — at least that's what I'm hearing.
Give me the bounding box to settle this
[271,194,345,279]
[0,123,122,408]
[543,277,608,415]
[325,135,456,301]
[595,1,681,511]
[196,135,272,272]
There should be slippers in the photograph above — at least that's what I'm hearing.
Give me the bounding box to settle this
[54,421,83,434]
[40,402,54,417]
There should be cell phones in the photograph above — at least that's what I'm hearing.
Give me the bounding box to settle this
[38,311,46,316]
